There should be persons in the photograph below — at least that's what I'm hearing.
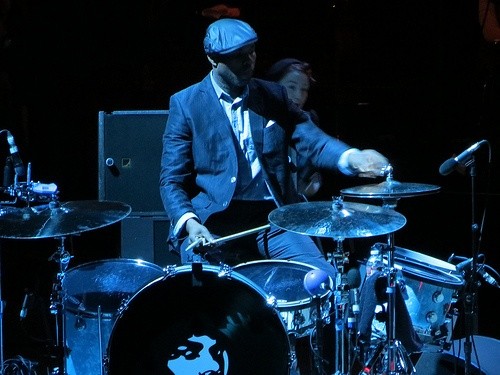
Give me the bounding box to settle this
[256,53,326,213]
[157,19,391,291]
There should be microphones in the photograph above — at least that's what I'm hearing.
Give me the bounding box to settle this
[439,139,487,175]
[456,256,475,270]
[7,131,26,177]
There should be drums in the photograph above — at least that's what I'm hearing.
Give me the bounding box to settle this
[52,257,165,375]
[229,258,334,338]
[356,243,467,351]
[102,263,297,375]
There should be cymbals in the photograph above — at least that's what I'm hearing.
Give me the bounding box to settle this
[267,198,407,238]
[338,178,441,199]
[0,198,133,241]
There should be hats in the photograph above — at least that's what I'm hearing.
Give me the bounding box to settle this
[203,18,258,55]
